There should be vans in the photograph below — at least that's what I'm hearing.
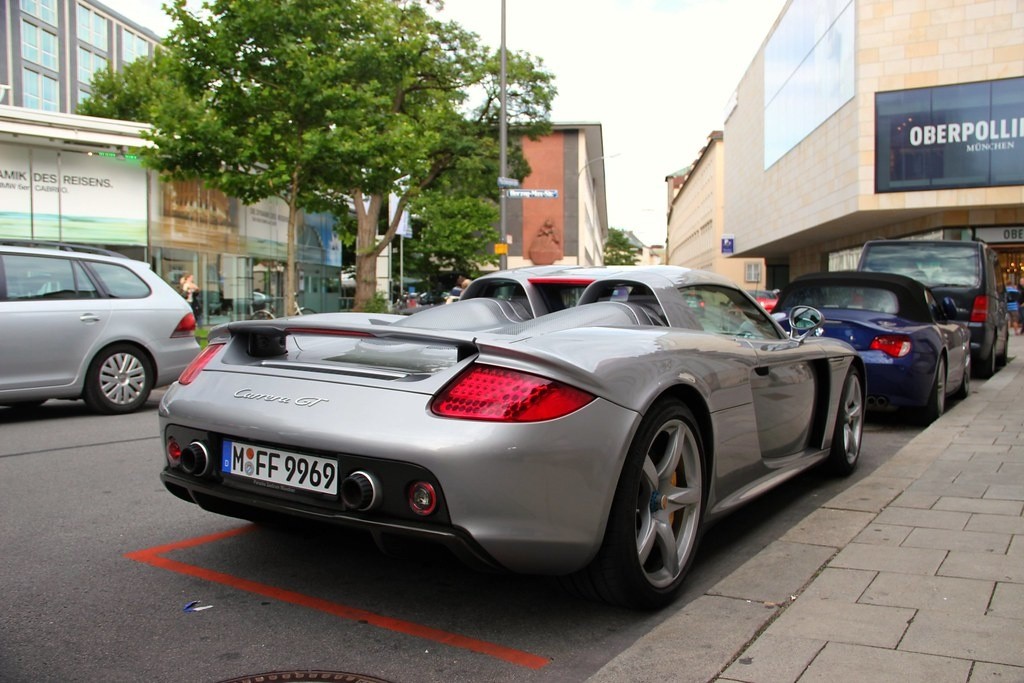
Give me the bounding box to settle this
[855,237,1018,378]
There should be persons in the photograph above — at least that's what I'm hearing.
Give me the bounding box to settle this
[1005,280,1020,335]
[1016,278,1024,335]
[181,274,200,323]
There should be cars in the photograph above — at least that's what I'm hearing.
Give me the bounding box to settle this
[197,289,225,316]
[682,293,707,317]
[764,270,973,425]
[719,289,780,321]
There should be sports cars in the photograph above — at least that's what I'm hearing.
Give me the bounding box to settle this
[159,265,865,610]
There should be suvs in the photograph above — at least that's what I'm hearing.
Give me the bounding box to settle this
[0,238,204,415]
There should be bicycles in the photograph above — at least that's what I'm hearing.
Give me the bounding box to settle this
[248,290,316,320]
